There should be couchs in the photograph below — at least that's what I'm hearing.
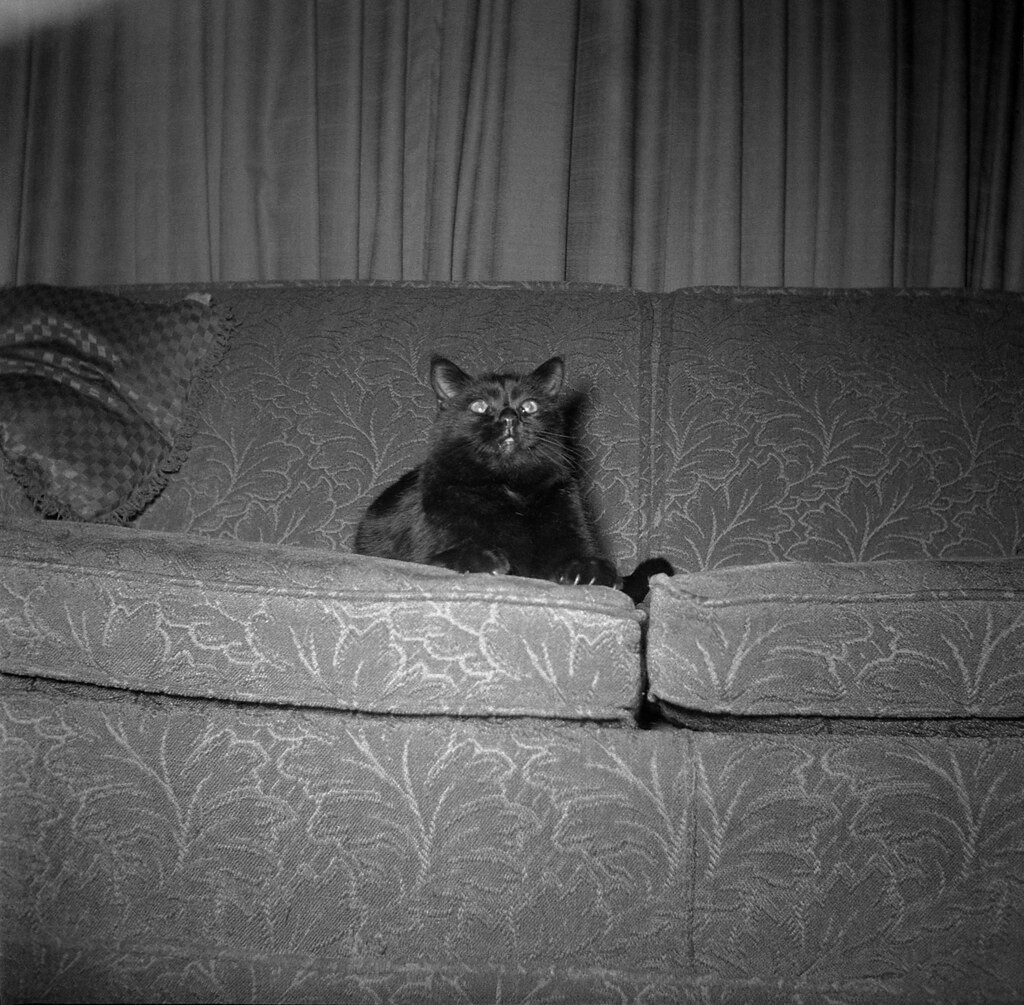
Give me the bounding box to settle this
[0,280,1024,1004]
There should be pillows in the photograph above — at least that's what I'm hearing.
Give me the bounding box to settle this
[0,284,229,522]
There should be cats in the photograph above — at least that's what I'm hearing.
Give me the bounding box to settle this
[353,351,674,604]
